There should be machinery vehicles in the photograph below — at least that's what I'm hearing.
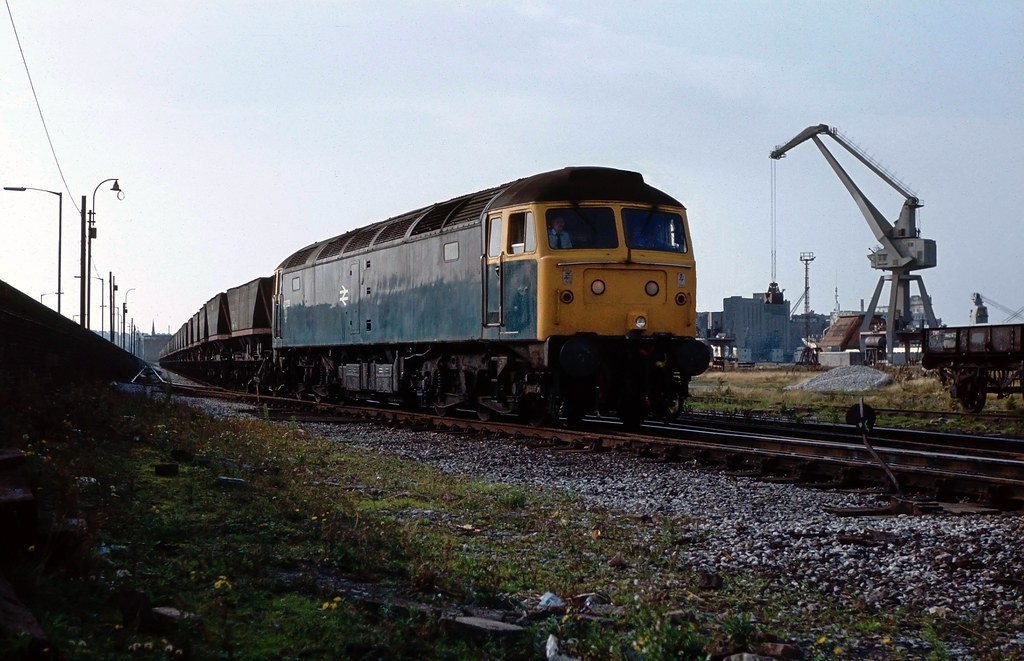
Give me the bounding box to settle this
[916,319,1024,415]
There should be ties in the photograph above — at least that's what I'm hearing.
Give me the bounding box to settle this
[557,233,562,249]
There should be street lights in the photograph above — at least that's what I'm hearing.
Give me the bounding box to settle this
[3,168,150,365]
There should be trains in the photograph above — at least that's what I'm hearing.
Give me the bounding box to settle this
[158,162,714,430]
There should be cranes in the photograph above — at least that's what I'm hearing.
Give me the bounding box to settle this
[763,121,951,367]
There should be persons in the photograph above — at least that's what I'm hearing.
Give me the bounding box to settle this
[547,214,573,249]
[628,222,677,250]
